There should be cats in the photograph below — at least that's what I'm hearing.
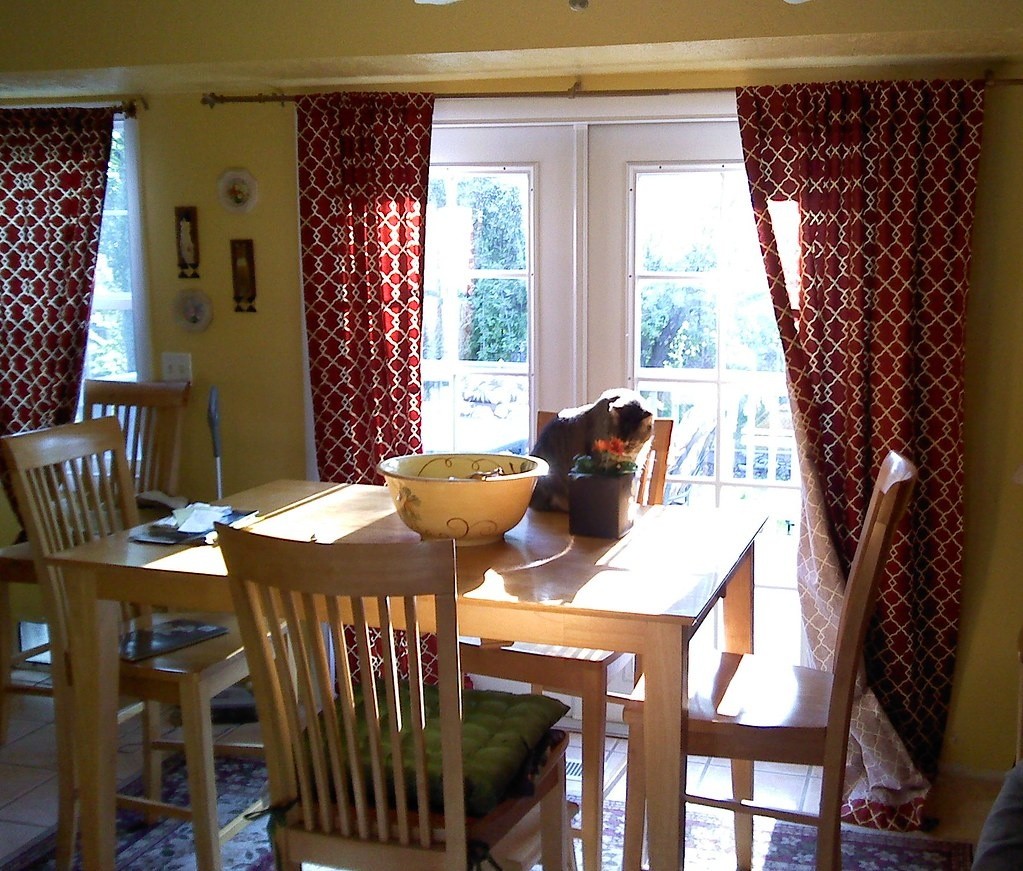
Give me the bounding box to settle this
[528,388,656,512]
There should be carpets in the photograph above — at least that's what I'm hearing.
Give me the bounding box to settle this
[1,745,975,871]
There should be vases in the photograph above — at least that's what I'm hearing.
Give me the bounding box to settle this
[569,473,634,539]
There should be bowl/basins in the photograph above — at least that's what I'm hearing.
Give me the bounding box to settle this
[377,454,548,545]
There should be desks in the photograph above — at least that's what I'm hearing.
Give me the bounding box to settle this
[44,479,769,871]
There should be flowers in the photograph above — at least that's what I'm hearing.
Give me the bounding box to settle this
[567,435,640,478]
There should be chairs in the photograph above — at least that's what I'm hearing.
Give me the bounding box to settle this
[1,378,917,871]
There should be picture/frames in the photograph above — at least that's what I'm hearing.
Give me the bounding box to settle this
[216,168,260,215]
[172,290,213,333]
